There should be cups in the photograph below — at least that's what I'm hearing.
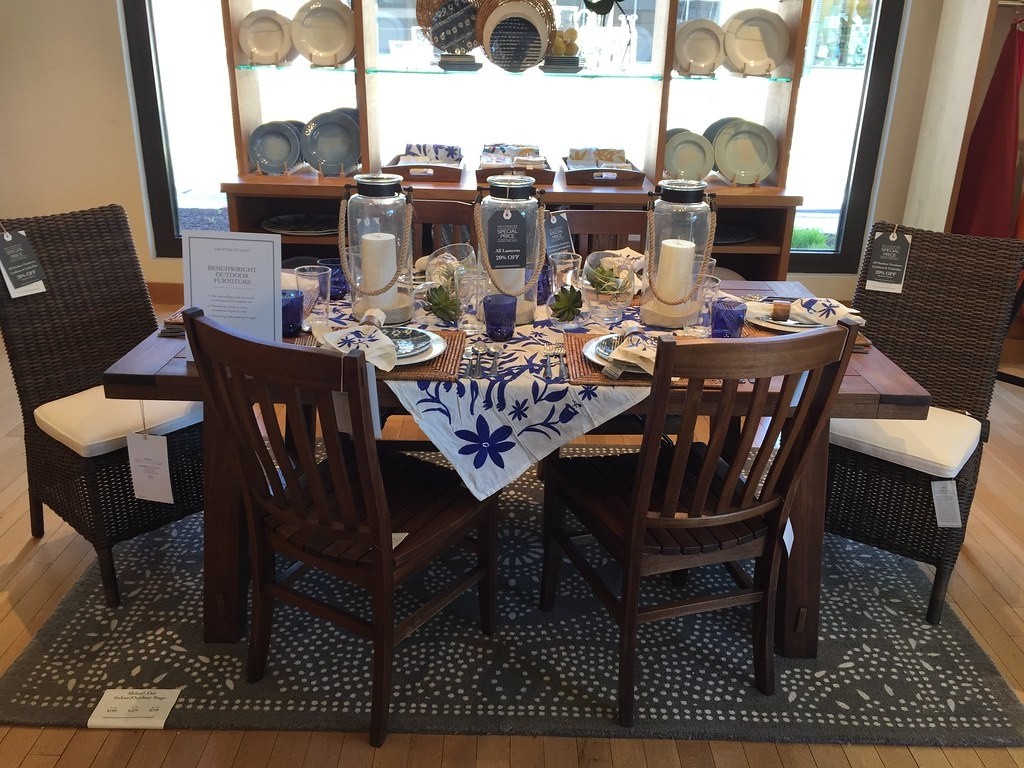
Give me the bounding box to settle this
[295,266,331,332]
[482,294,517,342]
[281,290,304,337]
[524,262,553,305]
[692,253,717,300]
[684,274,721,337]
[317,258,348,300]
[549,252,582,292]
[454,269,488,335]
[711,300,747,338]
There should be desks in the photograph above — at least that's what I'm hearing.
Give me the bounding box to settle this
[102,266,933,642]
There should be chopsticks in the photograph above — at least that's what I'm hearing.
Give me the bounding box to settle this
[852,345,869,353]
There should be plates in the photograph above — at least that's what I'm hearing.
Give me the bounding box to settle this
[248,121,306,174]
[239,9,300,62]
[300,108,360,176]
[744,301,825,333]
[291,0,355,65]
[439,54,482,71]
[562,273,620,293]
[379,327,432,357]
[384,326,447,365]
[664,9,790,185]
[583,334,647,373]
[538,56,583,73]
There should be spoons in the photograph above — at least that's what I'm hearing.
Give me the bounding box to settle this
[463,341,504,378]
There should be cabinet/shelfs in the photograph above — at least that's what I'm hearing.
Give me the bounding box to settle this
[220,0,812,281]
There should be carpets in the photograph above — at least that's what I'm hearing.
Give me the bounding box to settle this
[0,447,1024,746]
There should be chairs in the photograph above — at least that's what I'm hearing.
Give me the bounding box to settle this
[0,205,208,608]
[789,218,1024,624]
[184,306,502,747]
[411,199,478,268]
[538,320,859,725]
[544,209,648,270]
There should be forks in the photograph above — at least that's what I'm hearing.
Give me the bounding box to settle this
[554,339,568,379]
[544,342,554,379]
